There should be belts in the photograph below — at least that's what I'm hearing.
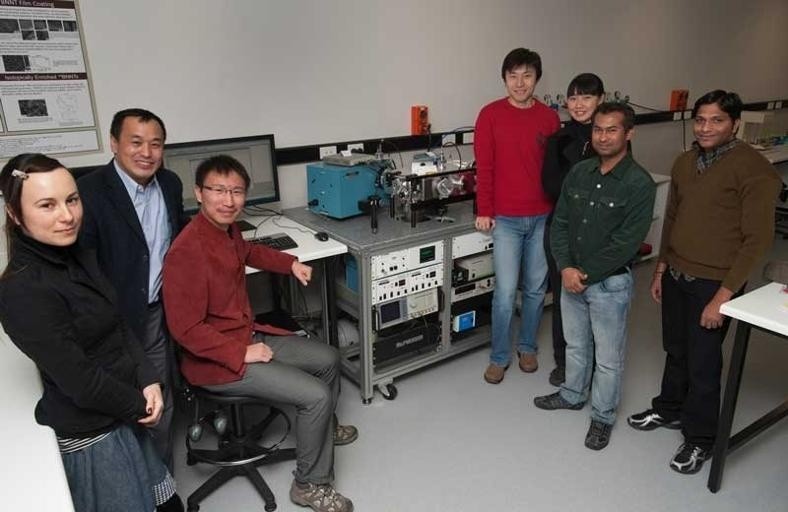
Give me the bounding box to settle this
[609,263,632,276]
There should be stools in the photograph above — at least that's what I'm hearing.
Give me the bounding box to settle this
[183,384,302,511]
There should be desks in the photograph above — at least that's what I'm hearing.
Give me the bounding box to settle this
[707,280,788,494]
[241,209,349,349]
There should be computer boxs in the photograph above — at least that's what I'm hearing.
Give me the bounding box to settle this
[255,308,307,338]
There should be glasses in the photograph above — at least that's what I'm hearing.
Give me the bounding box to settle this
[198,183,248,197]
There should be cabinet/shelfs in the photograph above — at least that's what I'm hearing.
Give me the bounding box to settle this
[638,172,672,261]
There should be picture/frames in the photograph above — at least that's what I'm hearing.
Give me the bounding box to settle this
[0,1,105,162]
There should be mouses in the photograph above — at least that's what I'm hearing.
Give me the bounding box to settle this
[314,232,328,241]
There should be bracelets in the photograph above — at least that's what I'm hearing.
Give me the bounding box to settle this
[652,270,666,274]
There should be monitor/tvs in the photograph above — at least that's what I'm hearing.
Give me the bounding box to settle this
[162,133,280,213]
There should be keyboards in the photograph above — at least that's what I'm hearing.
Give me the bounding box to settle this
[243,231,298,253]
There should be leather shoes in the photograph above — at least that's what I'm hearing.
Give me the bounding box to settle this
[519,352,538,372]
[484,363,504,384]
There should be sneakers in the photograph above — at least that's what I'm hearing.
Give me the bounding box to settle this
[550,365,565,386]
[627,408,681,431]
[333,424,357,445]
[669,441,713,474]
[289,479,353,512]
[584,420,612,450]
[534,391,584,410]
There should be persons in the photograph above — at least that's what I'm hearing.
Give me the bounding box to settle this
[0,153,184,511]
[626,88,784,475]
[542,73,633,387]
[473,47,561,383]
[533,101,656,450]
[162,154,359,512]
[74,108,183,479]
